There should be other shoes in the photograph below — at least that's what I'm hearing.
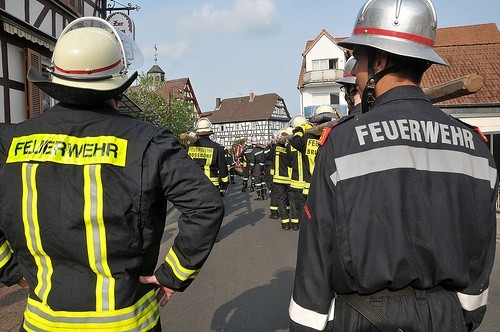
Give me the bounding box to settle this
[292,226,299,230]
[269,214,278,219]
[282,226,290,231]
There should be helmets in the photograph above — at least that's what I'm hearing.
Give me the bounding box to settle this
[278,115,307,138]
[195,117,214,135]
[336,56,357,85]
[338,0,450,68]
[27,15,144,109]
[310,105,338,122]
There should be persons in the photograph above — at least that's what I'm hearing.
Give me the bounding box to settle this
[217,146,236,197]
[328,55,362,128]
[237,128,295,231]
[292,105,338,228]
[288,1,499,332]
[186,117,230,242]
[0,18,225,332]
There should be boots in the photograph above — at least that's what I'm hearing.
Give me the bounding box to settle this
[249,183,255,192]
[254,189,267,200]
[242,182,248,192]
